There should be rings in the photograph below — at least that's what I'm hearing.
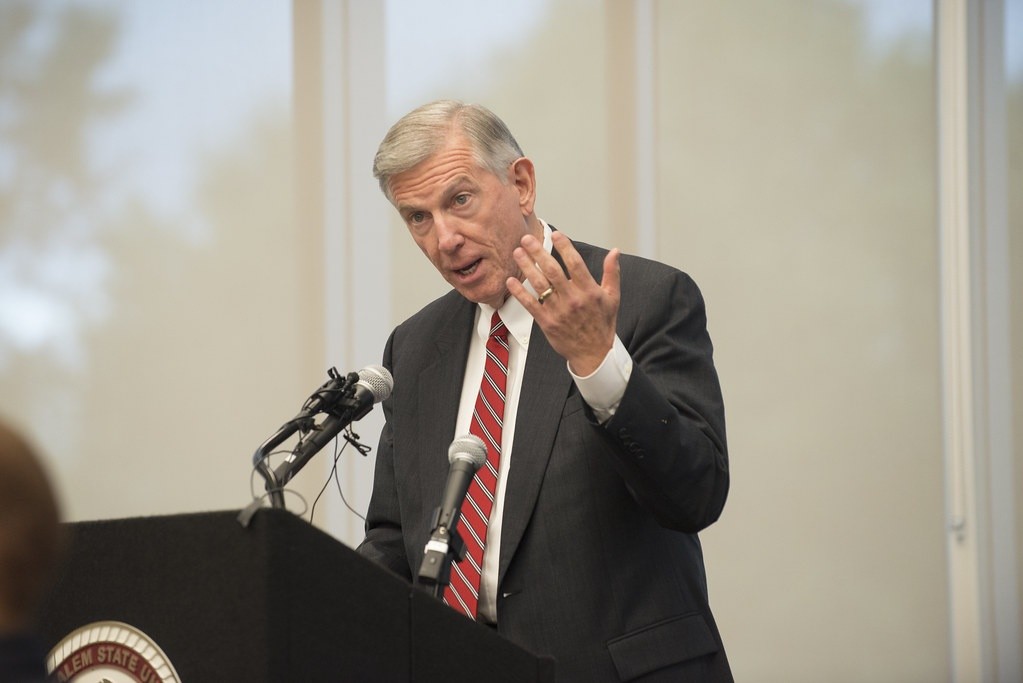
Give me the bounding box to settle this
[536,289,554,305]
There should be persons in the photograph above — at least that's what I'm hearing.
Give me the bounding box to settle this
[0,424,62,683]
[353,98,735,683]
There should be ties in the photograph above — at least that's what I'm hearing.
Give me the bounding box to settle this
[439,310,511,624]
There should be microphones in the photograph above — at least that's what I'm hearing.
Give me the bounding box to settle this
[265,366,393,493]
[417,434,488,585]
[341,372,359,393]
